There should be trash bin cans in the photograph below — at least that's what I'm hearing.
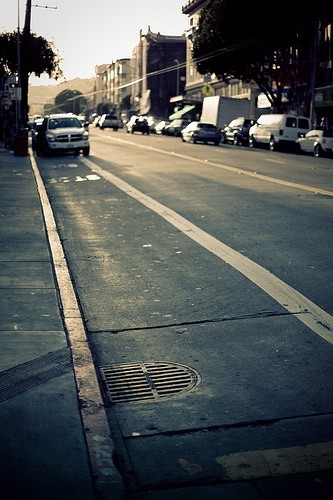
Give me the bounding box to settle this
[13,128,28,157]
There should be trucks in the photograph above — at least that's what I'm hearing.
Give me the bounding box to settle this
[198,96,251,133]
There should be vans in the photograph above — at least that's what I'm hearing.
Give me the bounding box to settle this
[247,113,313,153]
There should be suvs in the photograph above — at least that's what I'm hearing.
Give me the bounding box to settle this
[220,116,257,146]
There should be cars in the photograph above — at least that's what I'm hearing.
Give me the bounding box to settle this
[180,122,222,146]
[294,126,333,158]
[27,112,190,138]
[41,113,90,156]
